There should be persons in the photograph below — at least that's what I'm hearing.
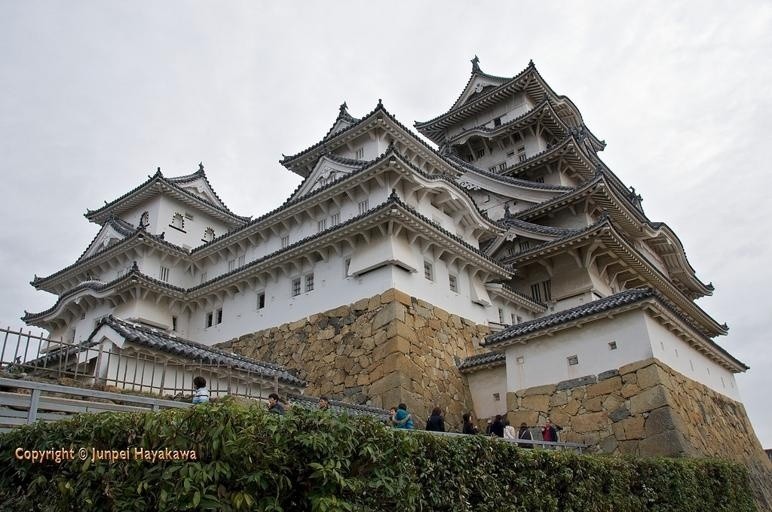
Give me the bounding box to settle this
[318,396,332,411]
[192,376,209,403]
[540,419,557,448]
[462,413,477,434]
[425,406,445,431]
[392,403,413,429]
[483,413,515,439]
[516,422,533,448]
[385,406,397,428]
[267,393,285,415]
